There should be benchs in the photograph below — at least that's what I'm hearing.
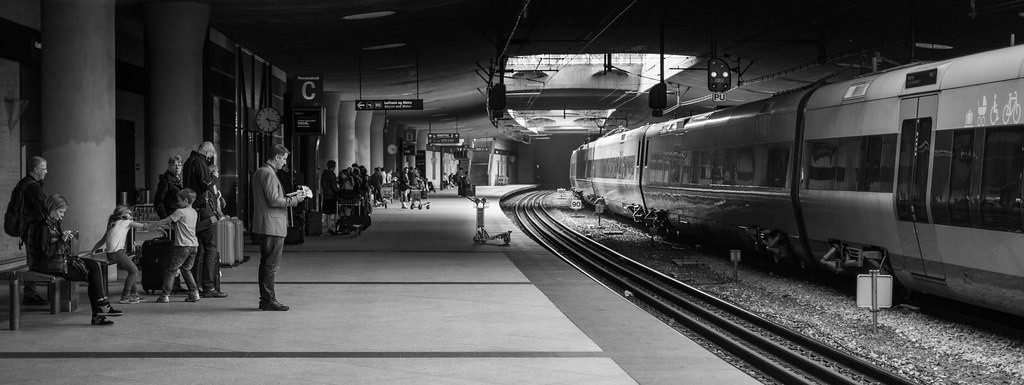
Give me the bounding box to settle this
[10,247,149,331]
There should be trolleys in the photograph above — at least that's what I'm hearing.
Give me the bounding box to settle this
[376,183,396,206]
[411,188,430,210]
[336,189,363,237]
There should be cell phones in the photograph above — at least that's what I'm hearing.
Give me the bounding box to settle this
[68,230,78,237]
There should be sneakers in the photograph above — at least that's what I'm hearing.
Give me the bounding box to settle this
[91,315,115,326]
[96,303,124,317]
[259,300,290,311]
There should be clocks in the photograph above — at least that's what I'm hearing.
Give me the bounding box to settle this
[255,108,281,132]
[387,144,398,155]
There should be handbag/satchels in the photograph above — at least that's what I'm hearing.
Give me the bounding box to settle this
[62,255,89,282]
[398,184,409,191]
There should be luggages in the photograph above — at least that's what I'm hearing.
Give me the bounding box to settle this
[212,215,246,267]
[142,235,176,294]
[305,209,323,236]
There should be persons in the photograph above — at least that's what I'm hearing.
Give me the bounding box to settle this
[321,159,372,237]
[3,156,123,326]
[90,206,148,304]
[443,173,456,189]
[142,141,227,303]
[457,167,471,198]
[253,143,308,311]
[368,166,436,207]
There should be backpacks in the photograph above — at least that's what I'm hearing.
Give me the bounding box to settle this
[3,180,37,250]
[25,217,49,258]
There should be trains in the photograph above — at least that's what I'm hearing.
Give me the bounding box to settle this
[570,45,1023,318]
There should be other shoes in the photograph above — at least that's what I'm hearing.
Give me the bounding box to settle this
[184,291,201,302]
[119,295,140,304]
[202,289,228,297]
[326,230,339,236]
[157,295,170,303]
[129,294,147,301]
